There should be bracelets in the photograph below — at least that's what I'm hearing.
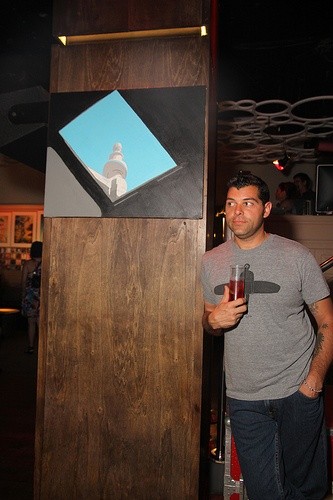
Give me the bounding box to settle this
[303,379,322,393]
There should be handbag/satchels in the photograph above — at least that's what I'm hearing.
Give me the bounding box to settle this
[31,261,41,288]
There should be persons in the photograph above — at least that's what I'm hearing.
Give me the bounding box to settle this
[274,181,300,215]
[20,241,42,353]
[200,171,333,500]
[292,173,316,216]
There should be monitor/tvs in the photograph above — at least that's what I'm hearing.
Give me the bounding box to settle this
[315,164,333,214]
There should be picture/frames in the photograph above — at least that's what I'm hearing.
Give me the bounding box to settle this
[37,210,44,242]
[12,212,36,247]
[0,212,11,247]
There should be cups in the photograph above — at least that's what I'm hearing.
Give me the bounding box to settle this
[229,265,246,307]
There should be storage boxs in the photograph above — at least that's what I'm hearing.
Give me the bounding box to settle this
[315,164,333,214]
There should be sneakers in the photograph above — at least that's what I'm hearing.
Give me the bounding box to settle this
[24,347,34,352]
